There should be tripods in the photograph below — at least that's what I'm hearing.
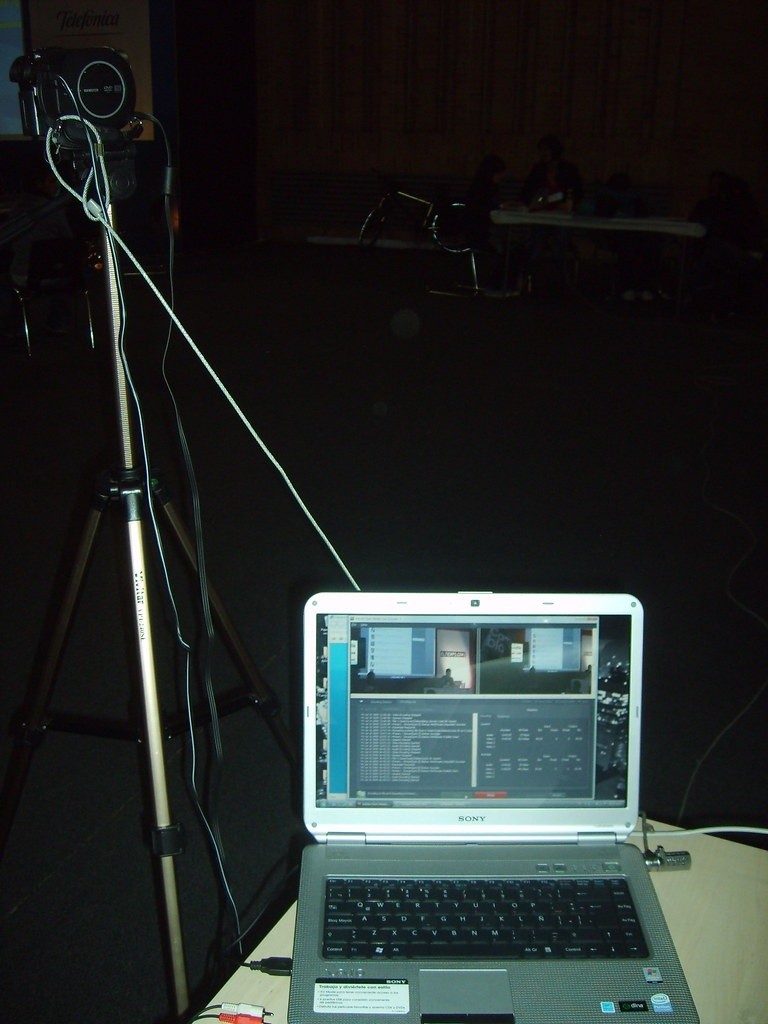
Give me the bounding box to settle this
[0,138,303,1024]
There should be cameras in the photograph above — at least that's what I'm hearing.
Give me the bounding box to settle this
[9,46,136,136]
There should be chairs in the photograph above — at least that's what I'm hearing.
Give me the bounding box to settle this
[0,199,96,358]
[424,184,481,299]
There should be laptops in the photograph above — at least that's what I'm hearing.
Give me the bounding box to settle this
[286,591,701,1024]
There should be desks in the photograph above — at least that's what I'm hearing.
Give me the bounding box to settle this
[191,817,768,1024]
[490,209,708,323]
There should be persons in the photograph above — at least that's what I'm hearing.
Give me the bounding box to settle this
[511,135,582,294]
[683,168,751,325]
[440,668,455,688]
[360,672,382,692]
[593,156,658,300]
[457,150,523,300]
